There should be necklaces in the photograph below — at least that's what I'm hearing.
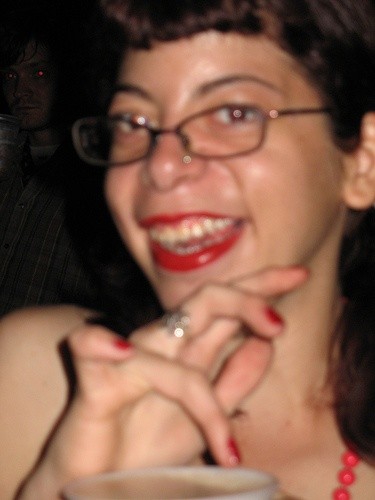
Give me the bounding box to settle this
[333,448,359,499]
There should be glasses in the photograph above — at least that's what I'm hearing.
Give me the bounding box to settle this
[70,98,328,166]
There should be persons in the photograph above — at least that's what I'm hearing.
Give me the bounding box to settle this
[0,0,375,499]
[0,14,163,339]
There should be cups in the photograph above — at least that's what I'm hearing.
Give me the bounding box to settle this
[66,467,272,499]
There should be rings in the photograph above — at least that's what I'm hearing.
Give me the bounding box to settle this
[163,308,198,342]
